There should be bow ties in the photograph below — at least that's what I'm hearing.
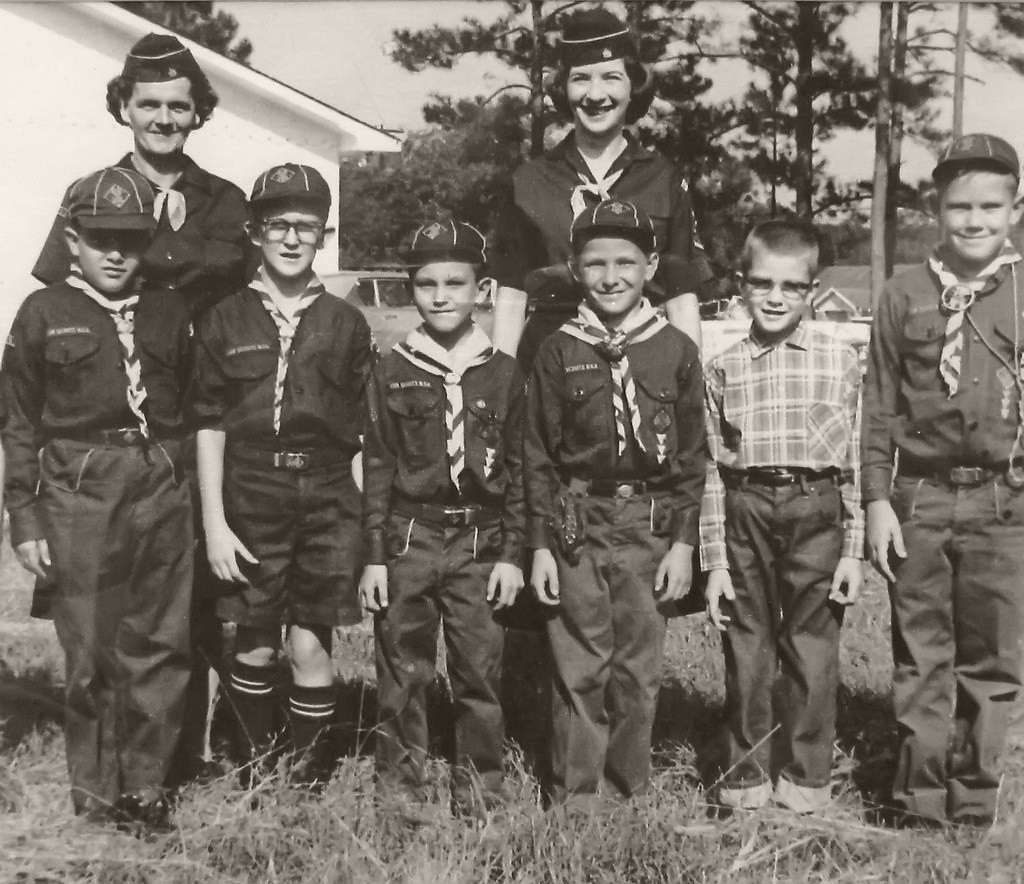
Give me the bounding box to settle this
[151,184,186,231]
[572,169,624,222]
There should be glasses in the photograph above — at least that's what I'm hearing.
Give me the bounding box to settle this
[262,218,325,244]
[743,275,810,301]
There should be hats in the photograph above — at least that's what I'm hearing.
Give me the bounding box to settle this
[572,200,657,236]
[398,219,488,265]
[932,133,1020,182]
[68,166,158,232]
[560,10,636,67]
[249,163,332,207]
[122,32,194,83]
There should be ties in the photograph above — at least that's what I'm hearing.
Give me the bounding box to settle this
[248,266,324,436]
[65,259,150,440]
[558,308,669,456]
[929,237,1023,402]
[389,320,500,495]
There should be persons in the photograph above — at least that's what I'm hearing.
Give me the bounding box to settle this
[188,162,379,805]
[356,220,526,826]
[698,220,865,823]
[489,10,716,758]
[861,134,1024,830]
[0,165,200,845]
[521,198,706,823]
[32,32,261,777]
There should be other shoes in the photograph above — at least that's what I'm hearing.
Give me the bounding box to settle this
[952,815,993,829]
[898,816,942,829]
[119,795,177,842]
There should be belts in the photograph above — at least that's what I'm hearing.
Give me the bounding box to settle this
[717,462,832,487]
[891,448,1024,485]
[560,472,673,498]
[227,439,350,473]
[53,427,174,447]
[391,495,504,528]
